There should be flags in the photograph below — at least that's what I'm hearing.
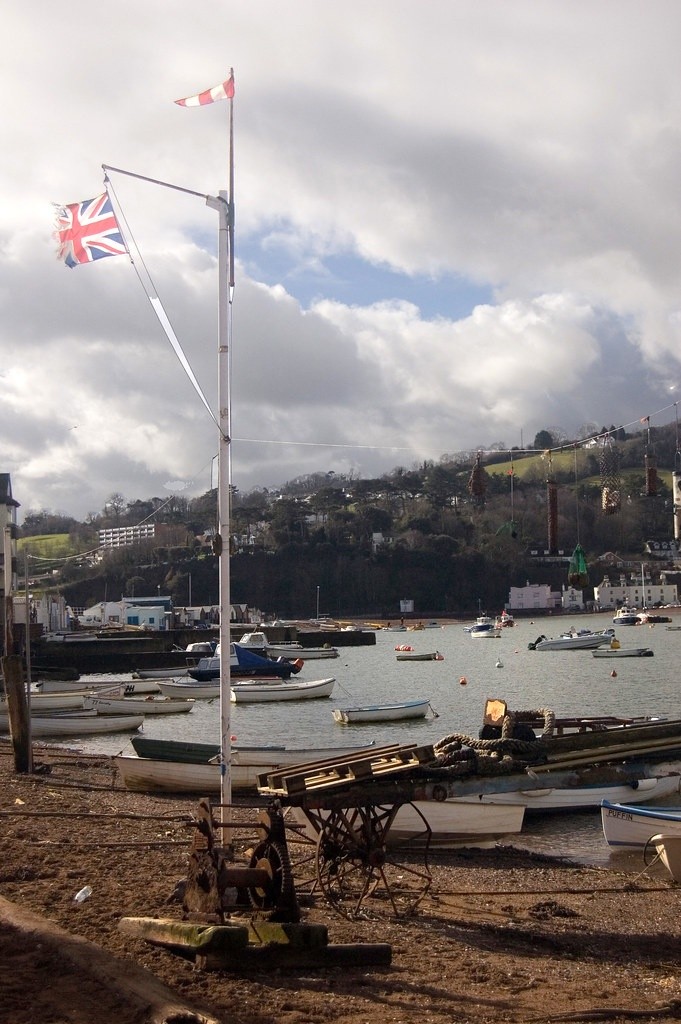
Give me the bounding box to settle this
[174,77,235,107]
[49,191,130,269]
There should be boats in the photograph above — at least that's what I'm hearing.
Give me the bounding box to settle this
[0,623,340,736]
[383,622,443,632]
[591,648,650,656]
[463,599,502,637]
[536,625,616,651]
[495,608,514,627]
[115,736,375,795]
[231,678,336,702]
[341,625,364,632]
[292,777,680,832]
[601,800,681,880]
[320,624,339,630]
[396,652,437,660]
[332,699,431,725]
[613,607,641,626]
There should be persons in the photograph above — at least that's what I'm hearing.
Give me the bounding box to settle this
[388,622,391,627]
[401,617,403,625]
[611,633,619,644]
